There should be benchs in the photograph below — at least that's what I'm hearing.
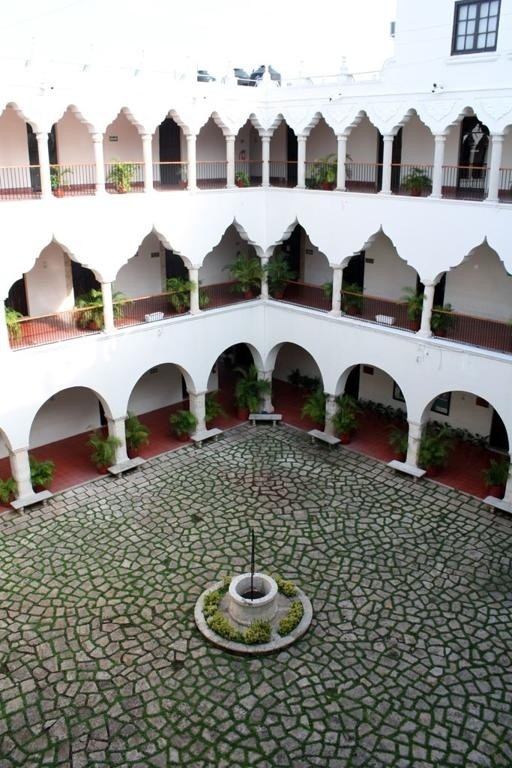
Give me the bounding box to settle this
[10,490,53,515]
[482,496,512,514]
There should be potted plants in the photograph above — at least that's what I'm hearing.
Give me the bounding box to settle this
[401,168,432,196]
[308,153,353,190]
[106,158,142,191]
[235,169,250,187]
[48,163,72,197]
[175,164,188,189]
